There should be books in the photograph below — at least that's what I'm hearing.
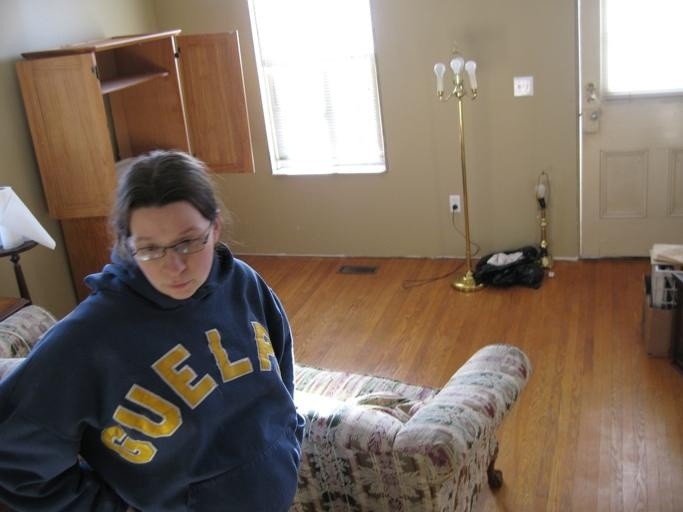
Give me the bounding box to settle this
[650,244,683,266]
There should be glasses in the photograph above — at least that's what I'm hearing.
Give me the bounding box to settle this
[130,219,212,260]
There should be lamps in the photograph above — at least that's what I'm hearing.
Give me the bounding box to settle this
[434,58,486,292]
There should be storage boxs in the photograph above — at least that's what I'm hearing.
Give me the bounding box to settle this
[641,274,674,358]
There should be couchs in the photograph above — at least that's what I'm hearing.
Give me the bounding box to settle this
[0,305,531,511]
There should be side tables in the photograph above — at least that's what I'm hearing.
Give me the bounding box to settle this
[0,295,30,321]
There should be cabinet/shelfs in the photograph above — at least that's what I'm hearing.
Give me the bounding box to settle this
[671,273,683,378]
[15,29,257,219]
[58,216,120,302]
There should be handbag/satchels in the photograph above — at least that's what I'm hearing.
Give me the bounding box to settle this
[473,246,544,289]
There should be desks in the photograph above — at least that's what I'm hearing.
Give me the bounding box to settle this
[0,240,38,306]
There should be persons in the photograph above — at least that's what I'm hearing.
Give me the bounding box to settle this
[0,151,305,512]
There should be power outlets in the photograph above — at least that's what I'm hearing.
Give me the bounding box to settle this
[534,171,554,270]
[448,195,461,212]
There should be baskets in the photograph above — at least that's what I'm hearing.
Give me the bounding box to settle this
[651,260,683,309]
